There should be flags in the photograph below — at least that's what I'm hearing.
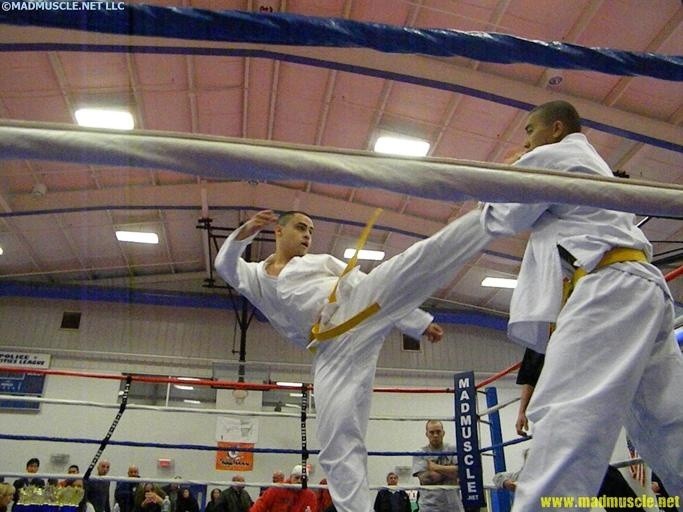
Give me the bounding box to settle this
[626,434,646,488]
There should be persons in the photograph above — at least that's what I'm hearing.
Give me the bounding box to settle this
[478,98,683,512]
[597,465,647,512]
[514,349,547,439]
[651,470,677,512]
[213,209,494,512]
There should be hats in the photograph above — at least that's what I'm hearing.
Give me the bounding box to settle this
[291,465,309,476]
